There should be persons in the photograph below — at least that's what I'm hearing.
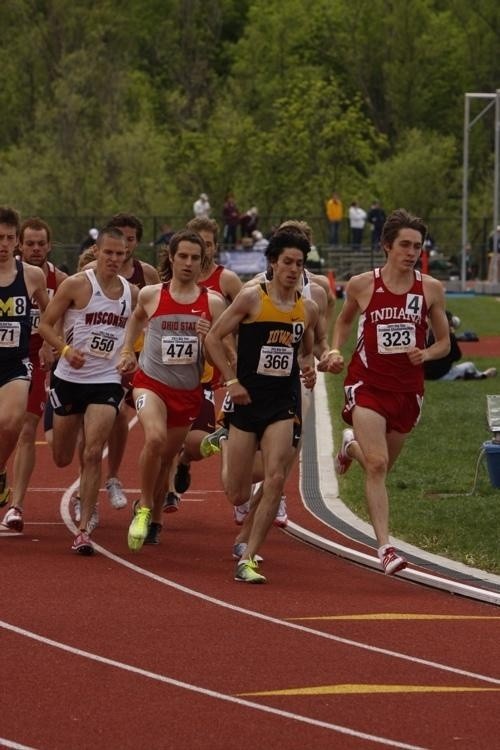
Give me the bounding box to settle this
[2,191,500,584]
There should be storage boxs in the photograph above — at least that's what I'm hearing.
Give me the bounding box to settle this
[482,440,500,488]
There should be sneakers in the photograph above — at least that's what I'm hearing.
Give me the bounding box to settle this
[255,480,289,528]
[127,503,154,553]
[144,521,164,546]
[333,427,358,475]
[73,493,84,522]
[233,560,268,584]
[199,426,229,458]
[161,490,181,513]
[0,468,10,509]
[105,477,128,510]
[70,530,96,555]
[173,459,192,494]
[1,504,26,533]
[232,486,254,525]
[375,543,411,578]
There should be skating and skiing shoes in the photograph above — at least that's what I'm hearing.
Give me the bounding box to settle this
[230,542,264,564]
